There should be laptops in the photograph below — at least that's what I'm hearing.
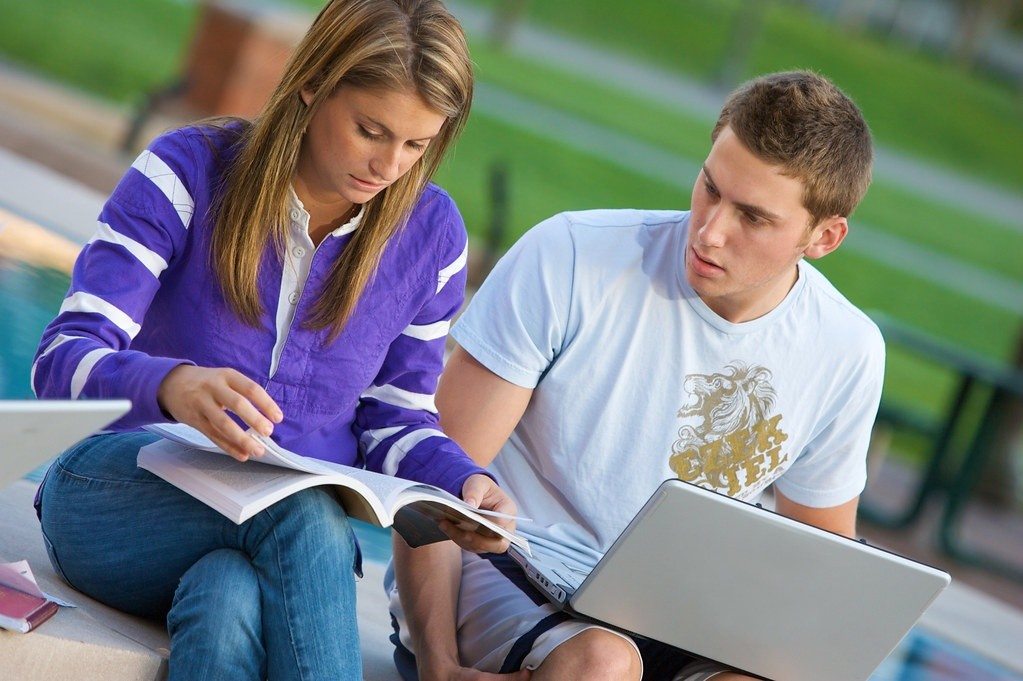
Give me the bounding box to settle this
[503,478,953,681]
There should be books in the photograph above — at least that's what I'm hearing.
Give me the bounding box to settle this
[0,581,58,634]
[135,420,536,558]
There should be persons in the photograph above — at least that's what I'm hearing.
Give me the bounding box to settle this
[381,71,886,681]
[32,0,518,681]
